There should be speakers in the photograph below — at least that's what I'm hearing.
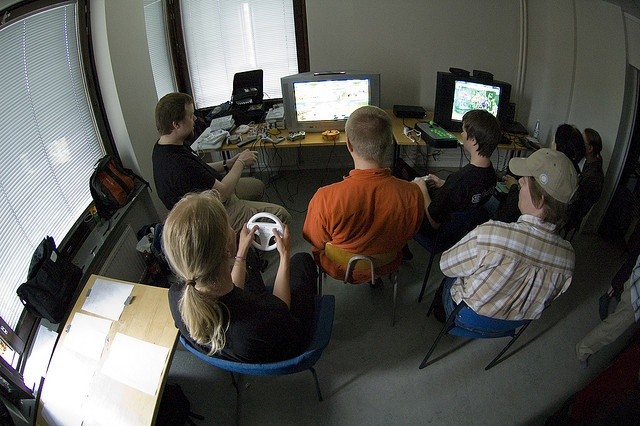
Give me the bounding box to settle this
[505,102,516,122]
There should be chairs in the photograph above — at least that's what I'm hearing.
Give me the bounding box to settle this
[414,210,479,298]
[419,276,531,372]
[179,296,335,400]
[317,249,405,327]
[565,201,594,245]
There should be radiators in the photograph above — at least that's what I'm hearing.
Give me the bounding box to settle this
[100,223,147,283]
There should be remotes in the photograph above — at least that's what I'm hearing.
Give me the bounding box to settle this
[237,137,257,148]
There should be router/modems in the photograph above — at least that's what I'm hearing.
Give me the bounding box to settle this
[393,105,427,119]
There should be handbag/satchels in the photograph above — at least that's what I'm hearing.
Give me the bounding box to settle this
[89,154,140,220]
[16,236,84,325]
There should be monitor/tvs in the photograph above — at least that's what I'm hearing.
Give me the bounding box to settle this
[281,72,380,133]
[433,71,512,133]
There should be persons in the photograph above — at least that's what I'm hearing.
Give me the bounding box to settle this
[496,124,588,224]
[562,128,604,233]
[574,254,640,370]
[152,92,293,272]
[599,219,640,322]
[302,106,425,286]
[161,189,319,364]
[433,147,580,339]
[409,110,500,255]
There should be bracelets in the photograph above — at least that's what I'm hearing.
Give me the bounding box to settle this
[223,161,229,171]
[234,257,246,260]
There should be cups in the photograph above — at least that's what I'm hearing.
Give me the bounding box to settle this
[84,215,99,234]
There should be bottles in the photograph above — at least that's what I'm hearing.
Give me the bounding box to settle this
[531,120,541,139]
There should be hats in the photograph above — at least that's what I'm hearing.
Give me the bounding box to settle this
[509,148,580,205]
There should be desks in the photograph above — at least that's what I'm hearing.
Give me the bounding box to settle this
[194,116,394,176]
[385,110,543,175]
[29,273,180,425]
